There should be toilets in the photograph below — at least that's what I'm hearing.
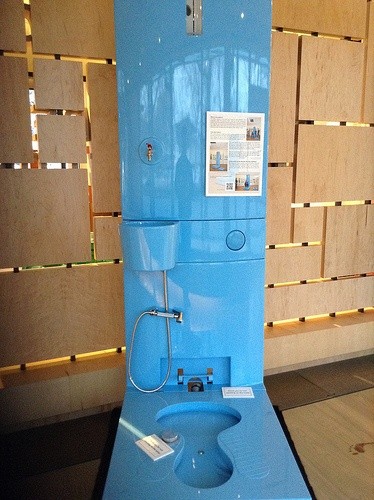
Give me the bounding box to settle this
[154,400,243,490]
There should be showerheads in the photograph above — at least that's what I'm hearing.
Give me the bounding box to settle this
[150,310,183,323]
